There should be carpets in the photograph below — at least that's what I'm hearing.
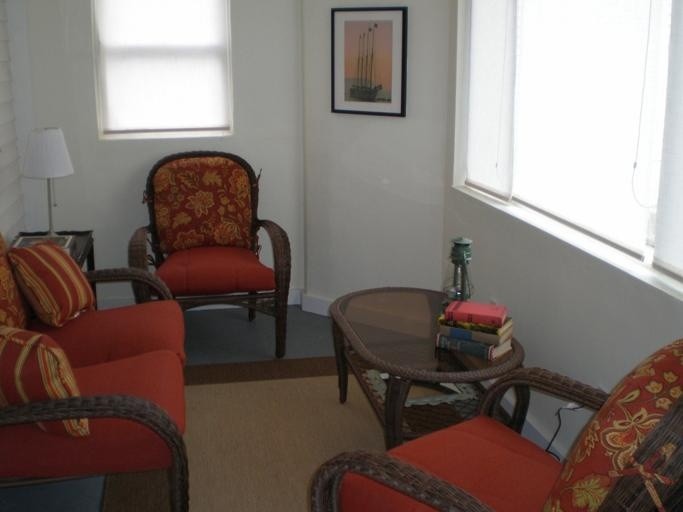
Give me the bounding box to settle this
[173,355,382,512]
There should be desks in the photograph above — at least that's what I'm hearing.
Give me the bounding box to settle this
[9,229,99,313]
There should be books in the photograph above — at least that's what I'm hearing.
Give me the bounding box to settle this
[434,300,515,361]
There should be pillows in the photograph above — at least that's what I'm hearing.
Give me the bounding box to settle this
[542,337,683,512]
[6,242,97,328]
[0,324,90,439]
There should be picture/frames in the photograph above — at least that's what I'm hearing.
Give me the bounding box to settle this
[329,5,408,119]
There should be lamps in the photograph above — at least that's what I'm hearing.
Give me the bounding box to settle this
[20,125,76,249]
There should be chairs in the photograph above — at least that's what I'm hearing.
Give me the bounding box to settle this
[128,147,292,361]
[307,367,683,512]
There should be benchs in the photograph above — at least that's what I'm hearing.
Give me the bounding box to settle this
[0,232,192,511]
